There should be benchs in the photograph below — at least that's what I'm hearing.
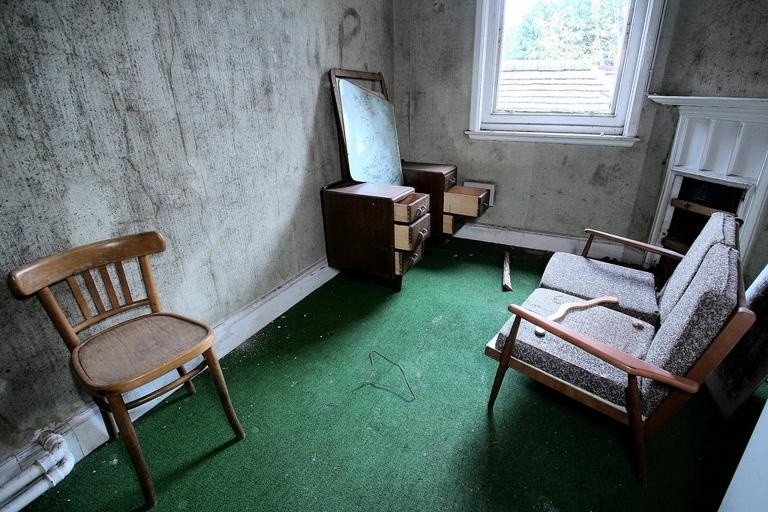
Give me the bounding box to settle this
[482,210,756,496]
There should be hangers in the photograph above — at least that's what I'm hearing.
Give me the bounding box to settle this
[534,295,620,338]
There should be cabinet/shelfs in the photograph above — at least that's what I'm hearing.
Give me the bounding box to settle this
[318,158,491,291]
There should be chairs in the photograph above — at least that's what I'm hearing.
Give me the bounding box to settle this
[9,231,247,505]
